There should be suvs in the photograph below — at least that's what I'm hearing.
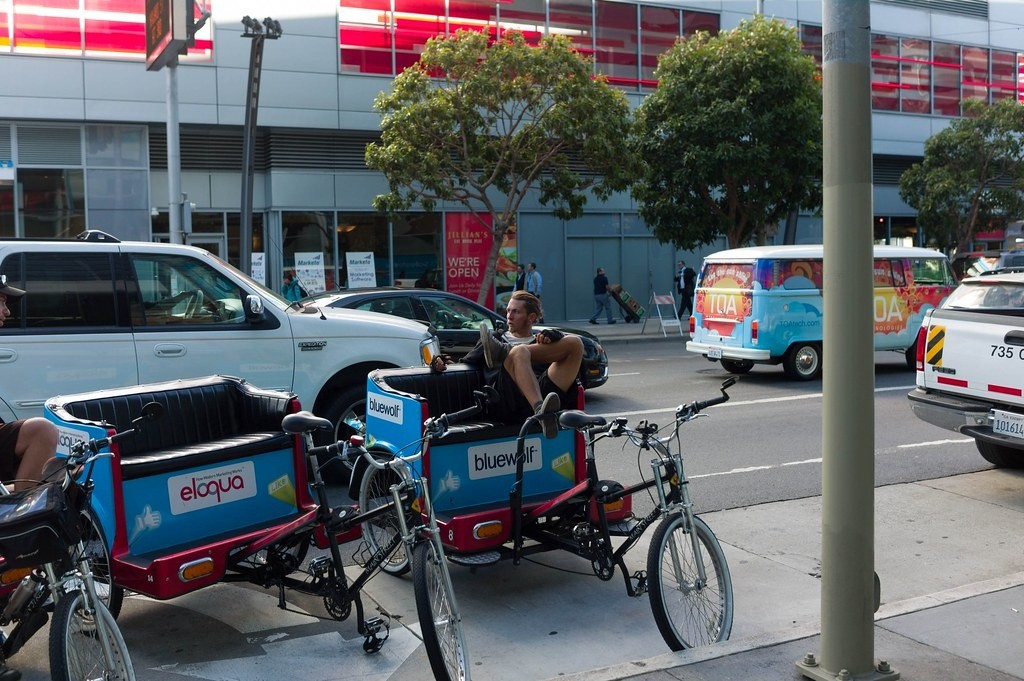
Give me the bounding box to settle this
[2,232,441,488]
[907,267,1024,466]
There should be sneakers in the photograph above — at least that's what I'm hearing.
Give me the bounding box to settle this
[0,630,23,681]
[536,392,561,439]
[480,321,514,369]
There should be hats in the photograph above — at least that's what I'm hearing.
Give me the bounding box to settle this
[0,274,27,297]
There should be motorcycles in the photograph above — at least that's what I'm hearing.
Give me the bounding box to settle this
[2,400,164,681]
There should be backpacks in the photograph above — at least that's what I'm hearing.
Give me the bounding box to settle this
[293,283,308,300]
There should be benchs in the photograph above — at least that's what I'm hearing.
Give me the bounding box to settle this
[379,366,583,448]
[59,375,296,479]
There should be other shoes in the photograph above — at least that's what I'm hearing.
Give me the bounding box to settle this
[538,318,544,324]
[674,315,682,322]
[608,320,616,324]
[688,316,692,320]
[589,319,599,324]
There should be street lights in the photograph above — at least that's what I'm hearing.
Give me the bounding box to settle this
[239,15,283,278]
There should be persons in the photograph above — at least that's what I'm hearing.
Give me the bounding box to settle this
[0,277,59,492]
[512,264,526,292]
[674,261,699,321]
[479,290,586,417]
[280,270,303,301]
[524,262,545,324]
[589,267,617,324]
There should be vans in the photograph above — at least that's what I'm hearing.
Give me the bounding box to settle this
[684,245,959,382]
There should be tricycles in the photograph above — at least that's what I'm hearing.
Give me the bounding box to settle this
[47,374,470,681]
[348,362,739,652]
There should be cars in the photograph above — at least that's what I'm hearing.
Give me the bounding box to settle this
[298,287,611,390]
